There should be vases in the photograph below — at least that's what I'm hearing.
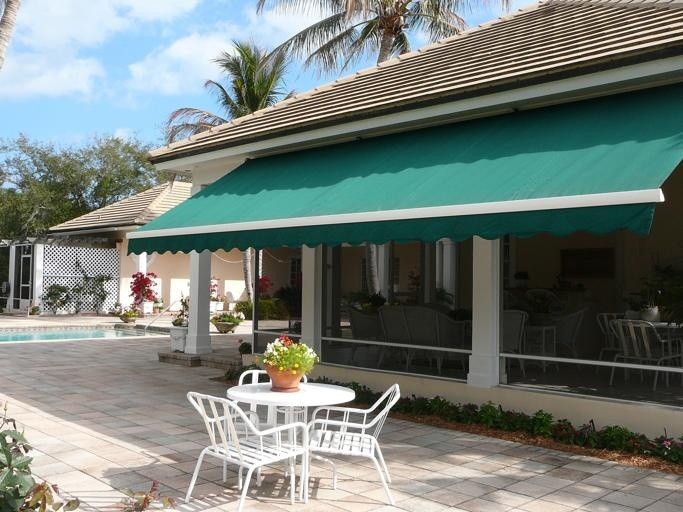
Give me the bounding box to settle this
[167,324,189,354]
[212,321,239,334]
[260,359,312,395]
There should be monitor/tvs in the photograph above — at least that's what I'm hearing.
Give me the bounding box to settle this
[558,246,617,281]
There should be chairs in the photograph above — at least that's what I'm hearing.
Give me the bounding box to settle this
[345,304,472,375]
[167,366,402,509]
[599,310,683,392]
[499,281,590,379]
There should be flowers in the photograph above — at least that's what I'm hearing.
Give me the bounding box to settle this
[108,265,250,330]
[250,332,320,377]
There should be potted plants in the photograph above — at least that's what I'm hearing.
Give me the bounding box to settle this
[274,283,302,318]
[365,294,387,314]
[634,280,662,322]
